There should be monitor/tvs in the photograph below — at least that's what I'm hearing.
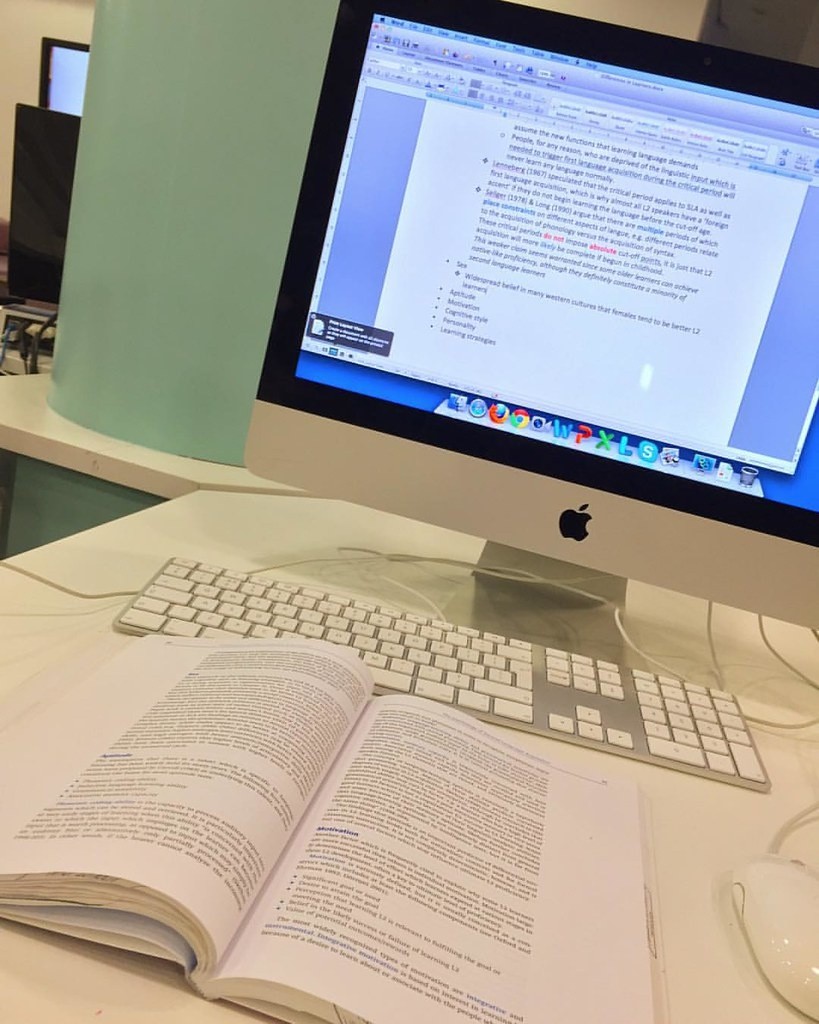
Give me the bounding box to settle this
[243,2,819,629]
[8,37,92,306]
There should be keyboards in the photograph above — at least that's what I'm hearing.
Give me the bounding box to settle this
[114,556,771,797]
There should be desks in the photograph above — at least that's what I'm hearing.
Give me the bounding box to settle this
[0,486,818,1024]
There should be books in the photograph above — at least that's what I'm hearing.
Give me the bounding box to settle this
[2,630,677,1023]
